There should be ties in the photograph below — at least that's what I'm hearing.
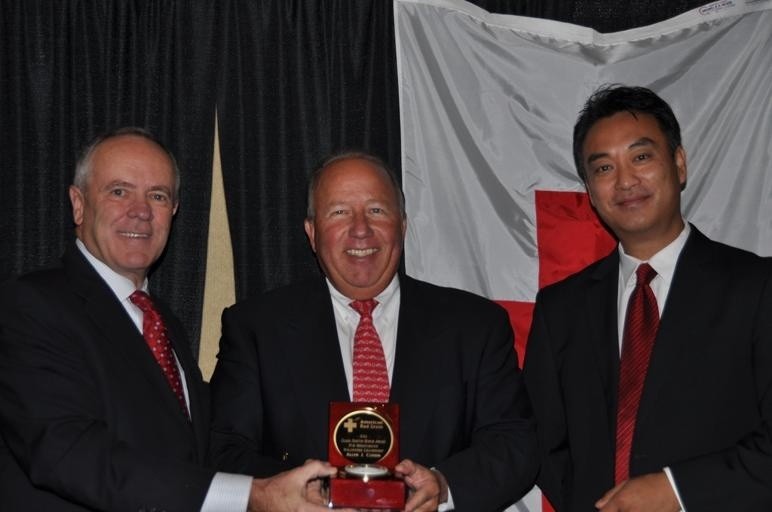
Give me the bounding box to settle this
[608,262,658,489]
[344,300,389,405]
[120,289,189,422]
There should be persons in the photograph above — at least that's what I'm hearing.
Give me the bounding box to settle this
[523,82,772,512]
[0,125,364,512]
[204,150,542,512]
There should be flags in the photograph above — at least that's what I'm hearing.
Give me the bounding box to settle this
[393,1,772,512]
